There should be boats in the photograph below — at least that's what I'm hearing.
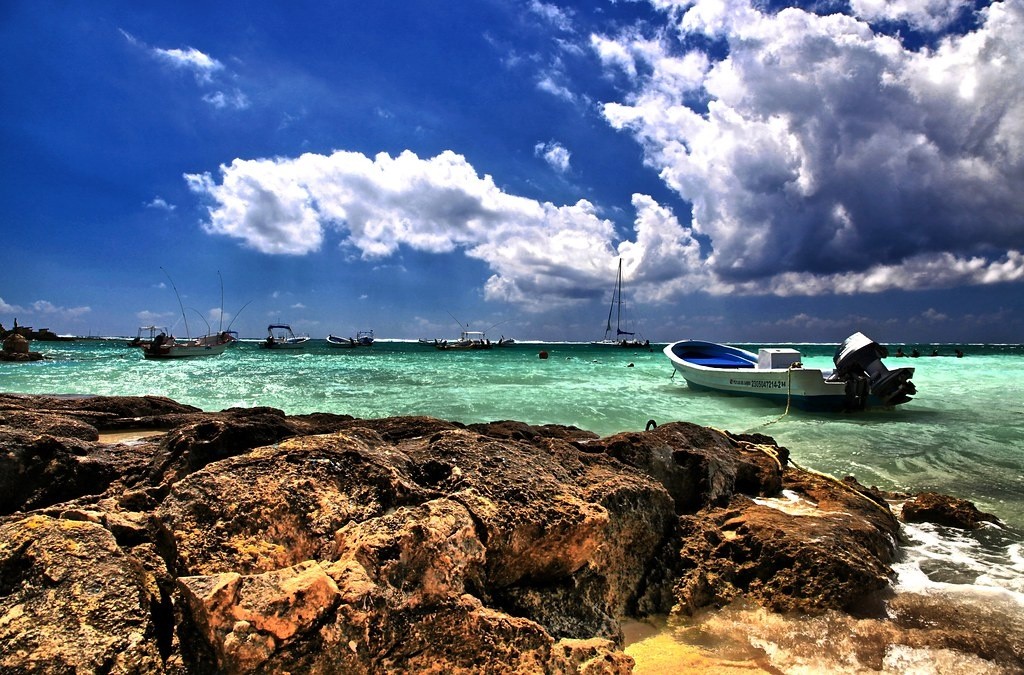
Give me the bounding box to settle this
[259,324,310,349]
[138,330,237,357]
[126,336,178,348]
[663,332,918,406]
[356,329,375,346]
[419,331,523,350]
[326,334,354,347]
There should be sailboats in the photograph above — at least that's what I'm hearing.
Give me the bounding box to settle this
[591,257,652,350]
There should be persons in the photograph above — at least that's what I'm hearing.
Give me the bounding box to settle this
[350,336,354,342]
[896,348,963,358]
[623,339,648,345]
[150,332,166,350]
[434,338,502,348]
